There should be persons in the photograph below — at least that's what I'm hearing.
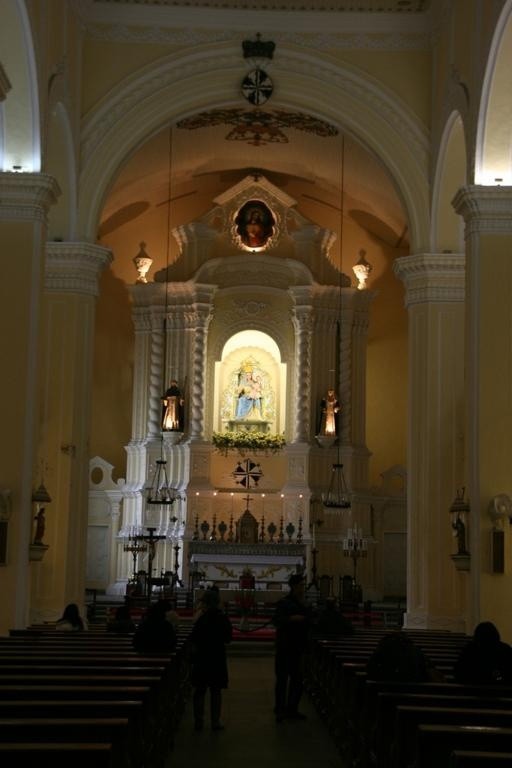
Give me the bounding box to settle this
[133,601,176,653]
[108,606,136,633]
[452,517,465,554]
[188,585,232,733]
[244,209,268,246]
[271,574,319,725]
[453,621,511,679]
[236,366,262,421]
[33,507,46,545]
[320,389,340,436]
[160,379,184,431]
[372,632,442,683]
[56,603,88,631]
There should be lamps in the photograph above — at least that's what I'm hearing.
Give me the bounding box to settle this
[318,135,351,511]
[140,125,184,505]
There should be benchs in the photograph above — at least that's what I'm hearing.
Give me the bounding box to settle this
[0,616,200,768]
[304,627,511,768]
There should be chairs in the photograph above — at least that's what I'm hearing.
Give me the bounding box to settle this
[124,571,178,603]
[316,574,362,609]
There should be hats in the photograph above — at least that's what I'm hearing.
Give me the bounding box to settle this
[197,590,220,608]
